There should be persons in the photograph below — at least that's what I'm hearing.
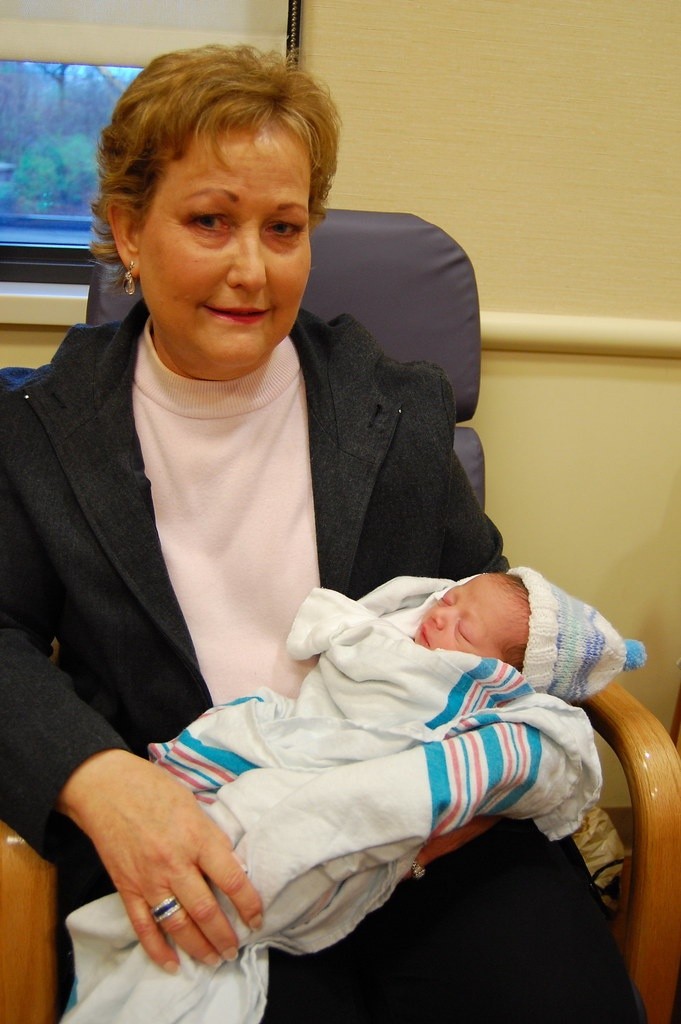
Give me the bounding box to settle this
[67,567,647,1024]
[0,46,653,1024]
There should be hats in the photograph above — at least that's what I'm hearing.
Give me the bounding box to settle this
[506,566,648,702]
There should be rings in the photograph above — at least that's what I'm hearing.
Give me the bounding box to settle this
[412,860,425,879]
[152,896,180,923]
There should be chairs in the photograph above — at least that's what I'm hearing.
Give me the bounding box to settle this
[2,212,681,1022]
